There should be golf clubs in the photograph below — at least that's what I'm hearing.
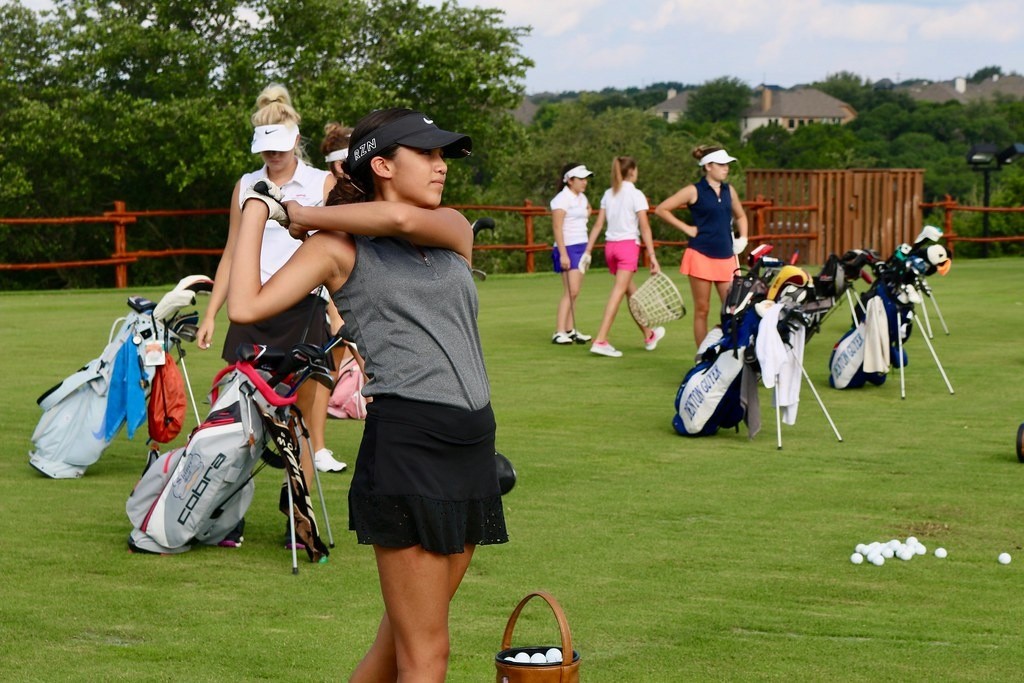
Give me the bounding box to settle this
[126,292,201,343]
[255,180,517,497]
[267,322,357,398]
[566,267,586,344]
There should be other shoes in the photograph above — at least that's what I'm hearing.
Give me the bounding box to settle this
[696,355,701,363]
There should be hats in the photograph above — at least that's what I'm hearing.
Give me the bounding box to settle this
[699,149,737,166]
[349,112,472,176]
[563,165,593,184]
[251,123,299,154]
[325,147,348,163]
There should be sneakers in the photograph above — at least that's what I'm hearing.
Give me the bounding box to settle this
[644,326,665,350]
[566,329,591,341]
[315,448,347,472]
[590,341,623,357]
[284,519,306,550]
[552,332,572,344]
[219,519,244,547]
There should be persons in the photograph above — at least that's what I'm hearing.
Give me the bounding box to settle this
[577,156,665,358]
[228,111,508,683]
[654,144,748,350]
[310,120,374,473]
[195,83,338,551]
[548,162,592,345]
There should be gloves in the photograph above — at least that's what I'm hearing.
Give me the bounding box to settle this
[241,177,291,229]
[733,236,748,254]
[578,254,592,274]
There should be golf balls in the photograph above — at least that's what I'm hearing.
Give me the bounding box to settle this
[504,648,564,663]
[998,553,1012,564]
[934,548,947,558]
[850,536,926,566]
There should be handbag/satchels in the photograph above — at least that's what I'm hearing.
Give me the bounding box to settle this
[148,353,186,444]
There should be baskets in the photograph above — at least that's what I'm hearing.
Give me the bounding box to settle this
[628,271,686,328]
